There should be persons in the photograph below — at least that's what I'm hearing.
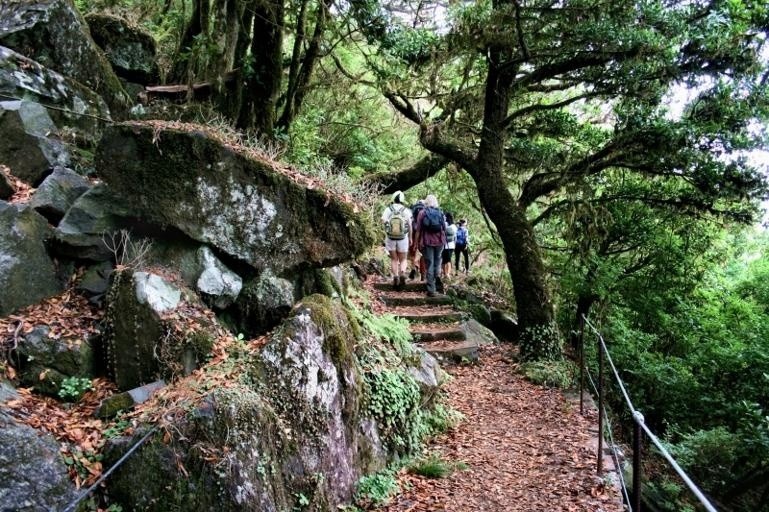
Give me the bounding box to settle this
[454,219,472,277]
[379,189,414,289]
[408,199,428,281]
[438,212,458,281]
[411,193,448,297]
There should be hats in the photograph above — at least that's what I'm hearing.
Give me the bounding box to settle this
[391,191,404,202]
[445,212,453,217]
[424,195,438,208]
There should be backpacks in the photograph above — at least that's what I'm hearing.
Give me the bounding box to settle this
[385,211,409,240]
[413,204,424,220]
[456,227,464,244]
[423,208,443,233]
[446,228,455,241]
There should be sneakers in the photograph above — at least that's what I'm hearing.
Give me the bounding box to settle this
[446,274,451,281]
[409,269,415,279]
[394,276,407,286]
[435,277,441,286]
[427,292,436,297]
